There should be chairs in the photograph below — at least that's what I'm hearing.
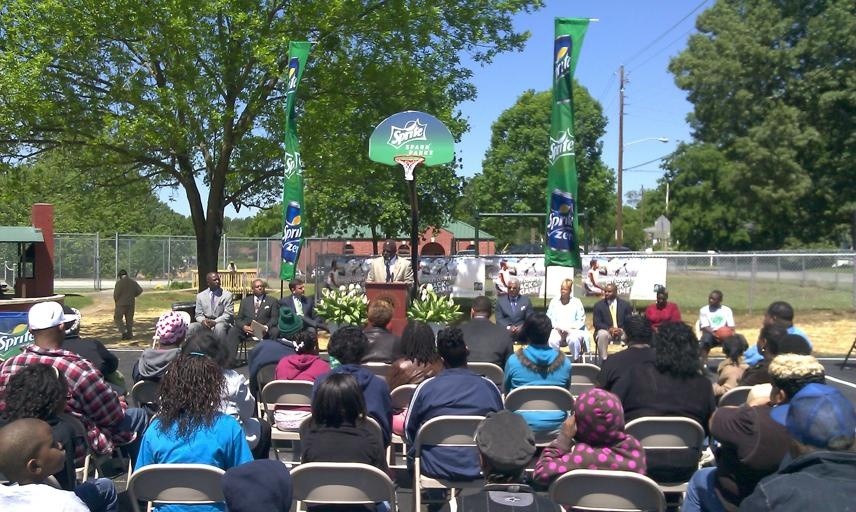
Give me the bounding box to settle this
[59,300,752,512]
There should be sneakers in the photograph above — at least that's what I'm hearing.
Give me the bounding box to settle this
[120,332,133,341]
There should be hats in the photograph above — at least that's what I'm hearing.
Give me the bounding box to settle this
[472,409,537,474]
[276,305,304,337]
[154,308,192,346]
[27,300,82,337]
[768,382,855,453]
[218,457,294,512]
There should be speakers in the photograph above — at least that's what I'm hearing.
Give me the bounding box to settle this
[171,301,197,323]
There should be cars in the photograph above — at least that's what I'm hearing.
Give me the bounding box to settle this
[830,259,853,269]
[453,239,631,255]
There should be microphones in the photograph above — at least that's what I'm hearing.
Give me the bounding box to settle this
[384,259,390,273]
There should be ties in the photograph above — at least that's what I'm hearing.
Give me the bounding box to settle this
[210,293,217,311]
[510,298,516,311]
[610,302,617,328]
[295,298,304,317]
[384,261,392,283]
[254,297,261,318]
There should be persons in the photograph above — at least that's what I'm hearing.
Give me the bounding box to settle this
[699,290,735,361]
[113,272,143,340]
[545,280,589,363]
[0,296,855,510]
[366,244,418,287]
[277,280,321,355]
[646,289,681,346]
[225,280,280,369]
[184,272,235,341]
[593,284,633,364]
[495,278,534,346]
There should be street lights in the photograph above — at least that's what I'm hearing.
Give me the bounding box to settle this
[615,137,671,248]
